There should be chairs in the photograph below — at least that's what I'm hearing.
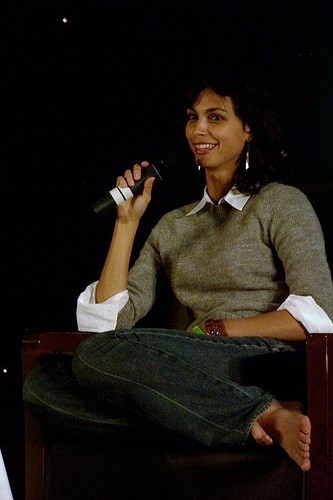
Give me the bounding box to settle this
[23,326,332,499]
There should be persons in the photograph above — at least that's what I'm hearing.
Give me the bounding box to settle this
[23,67,333,472]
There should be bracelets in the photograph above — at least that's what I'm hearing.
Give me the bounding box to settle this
[192,318,228,336]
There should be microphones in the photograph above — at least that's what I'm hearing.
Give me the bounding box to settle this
[92,162,169,214]
